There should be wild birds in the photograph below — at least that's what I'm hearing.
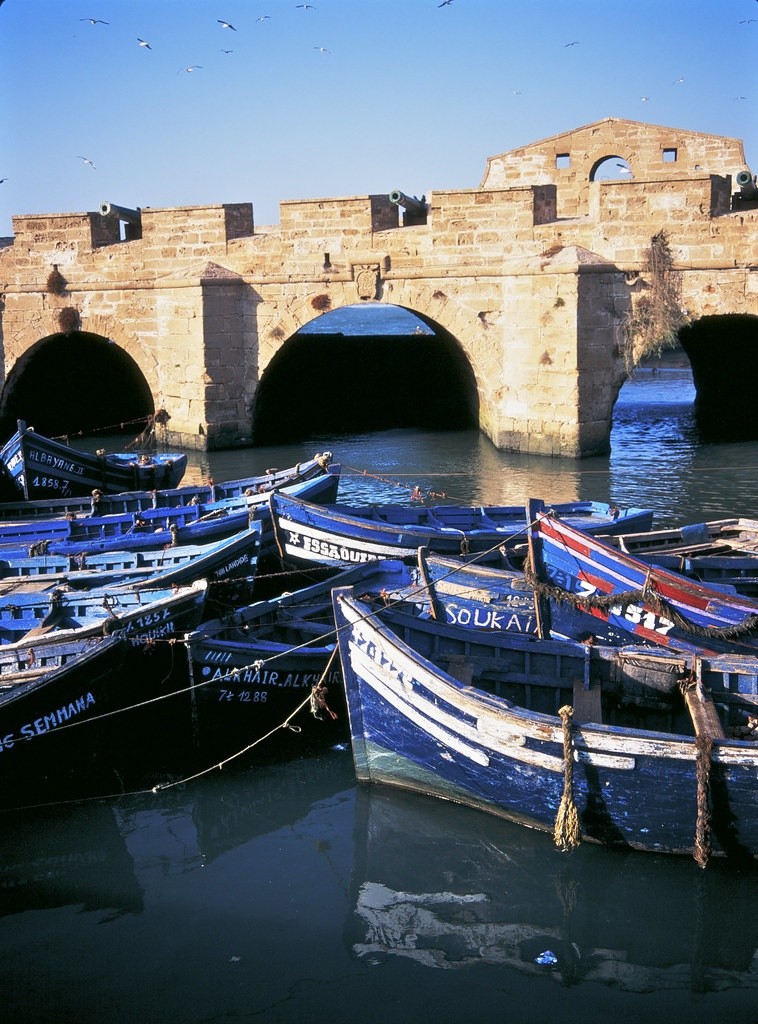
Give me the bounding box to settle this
[511,89,521,96]
[640,77,685,104]
[313,46,332,54]
[215,16,274,54]
[437,0,454,8]
[136,37,153,50]
[79,18,110,25]
[77,155,97,170]
[732,96,747,103]
[737,19,757,25]
[563,42,579,48]
[295,2,315,11]
[177,64,204,76]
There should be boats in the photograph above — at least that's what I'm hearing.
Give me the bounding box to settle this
[418,518,758,616]
[523,497,758,658]
[331,583,758,863]
[272,488,654,595]
[0,451,342,770]
[0,419,189,501]
[184,555,430,770]
